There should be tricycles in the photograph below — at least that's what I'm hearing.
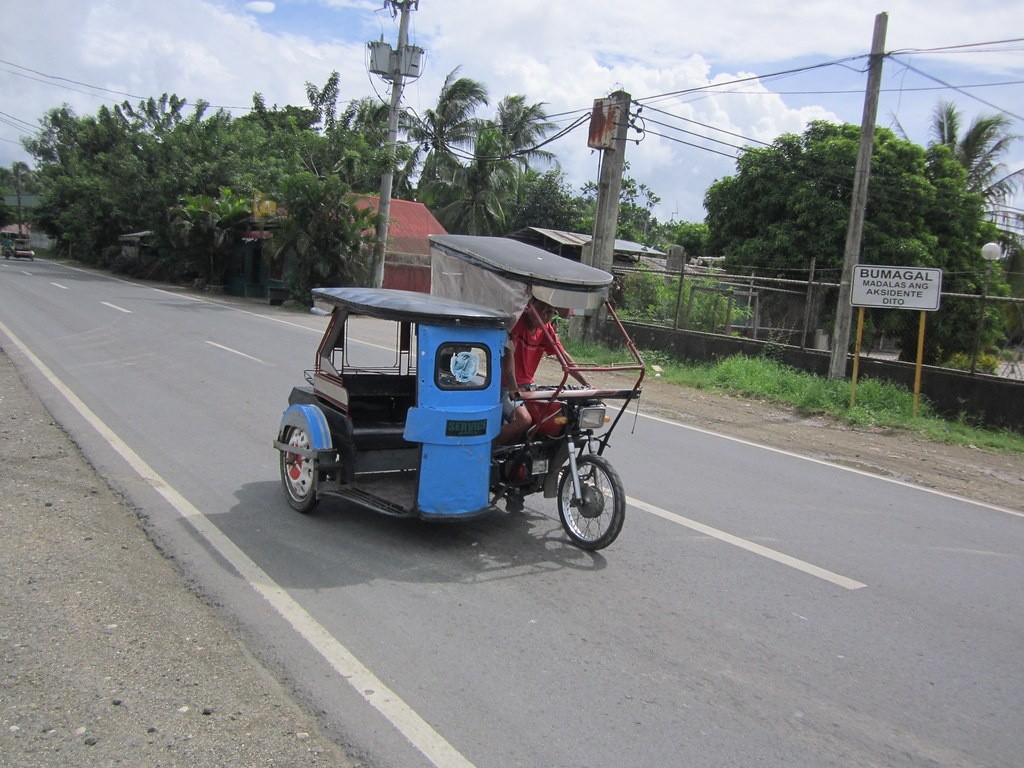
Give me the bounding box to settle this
[281,232,653,552]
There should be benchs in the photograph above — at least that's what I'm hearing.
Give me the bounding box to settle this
[344,391,420,450]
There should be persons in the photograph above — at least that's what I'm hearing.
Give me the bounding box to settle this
[494,298,605,447]
[2,237,14,253]
